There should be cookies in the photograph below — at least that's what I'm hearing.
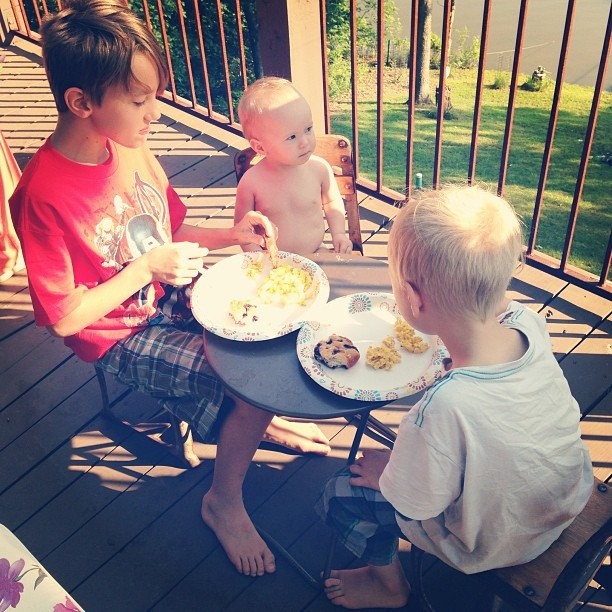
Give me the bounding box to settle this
[314,332,359,368]
[230,297,260,325]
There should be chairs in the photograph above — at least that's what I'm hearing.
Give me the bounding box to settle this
[420,474,612,612]
[235,135,365,255]
[95,363,200,467]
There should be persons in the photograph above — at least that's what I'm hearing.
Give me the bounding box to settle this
[7,1,330,579]
[318,183,596,610]
[232,76,354,256]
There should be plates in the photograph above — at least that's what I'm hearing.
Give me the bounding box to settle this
[295,291,452,402]
[189,249,331,343]
